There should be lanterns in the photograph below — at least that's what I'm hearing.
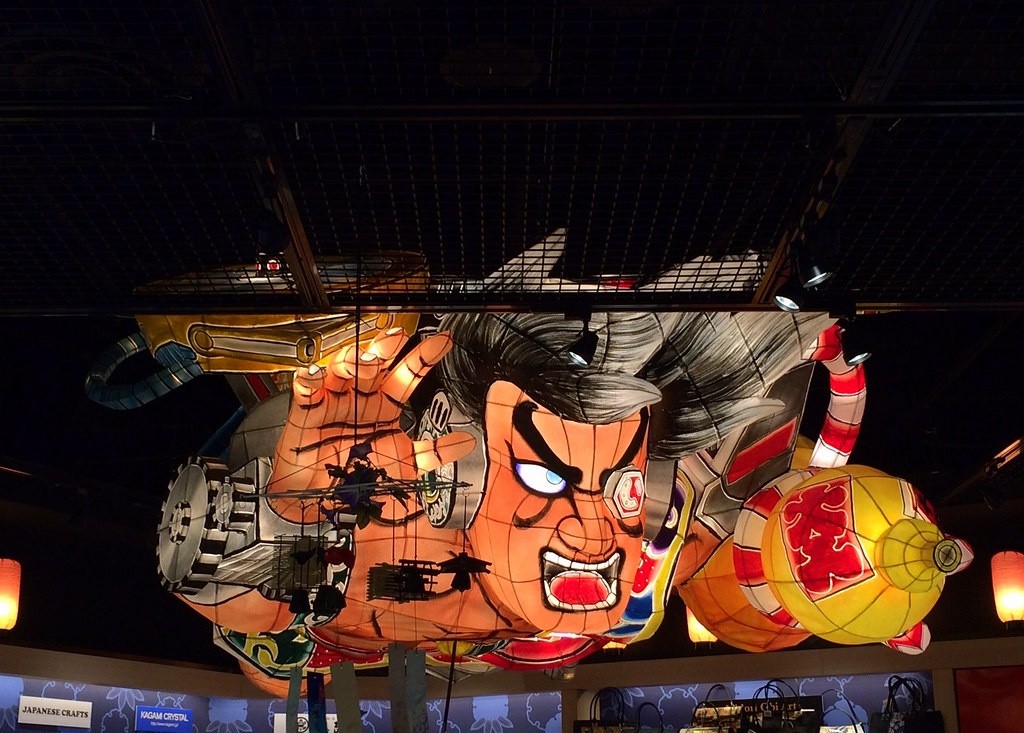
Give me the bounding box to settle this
[992,550,1024,622]
[0,559,21,629]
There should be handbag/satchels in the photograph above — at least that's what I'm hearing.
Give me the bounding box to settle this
[573,687,635,733]
[740,679,804,733]
[815,689,866,733]
[677,700,734,733]
[870,675,943,733]
[693,684,742,728]
[621,702,678,733]
